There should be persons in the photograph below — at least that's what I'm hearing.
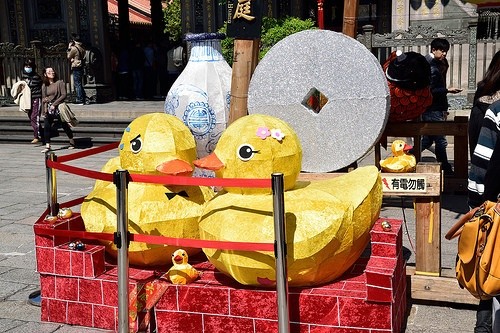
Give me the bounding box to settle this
[466,50,500,333]
[166,43,186,89]
[66,32,86,105]
[18,58,43,144]
[40,66,75,153]
[408,37,454,178]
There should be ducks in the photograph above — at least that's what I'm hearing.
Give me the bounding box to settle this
[78,112,416,289]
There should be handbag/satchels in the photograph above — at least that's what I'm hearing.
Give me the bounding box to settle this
[445,201,500,300]
[48,103,59,116]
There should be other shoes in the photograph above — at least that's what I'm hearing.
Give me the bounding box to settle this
[73,100,90,106]
[41,148,50,154]
[68,143,74,149]
[443,170,454,175]
[31,139,38,144]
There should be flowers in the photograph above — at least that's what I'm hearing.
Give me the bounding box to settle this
[256,126,285,141]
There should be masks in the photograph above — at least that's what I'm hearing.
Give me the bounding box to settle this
[24,68,32,73]
